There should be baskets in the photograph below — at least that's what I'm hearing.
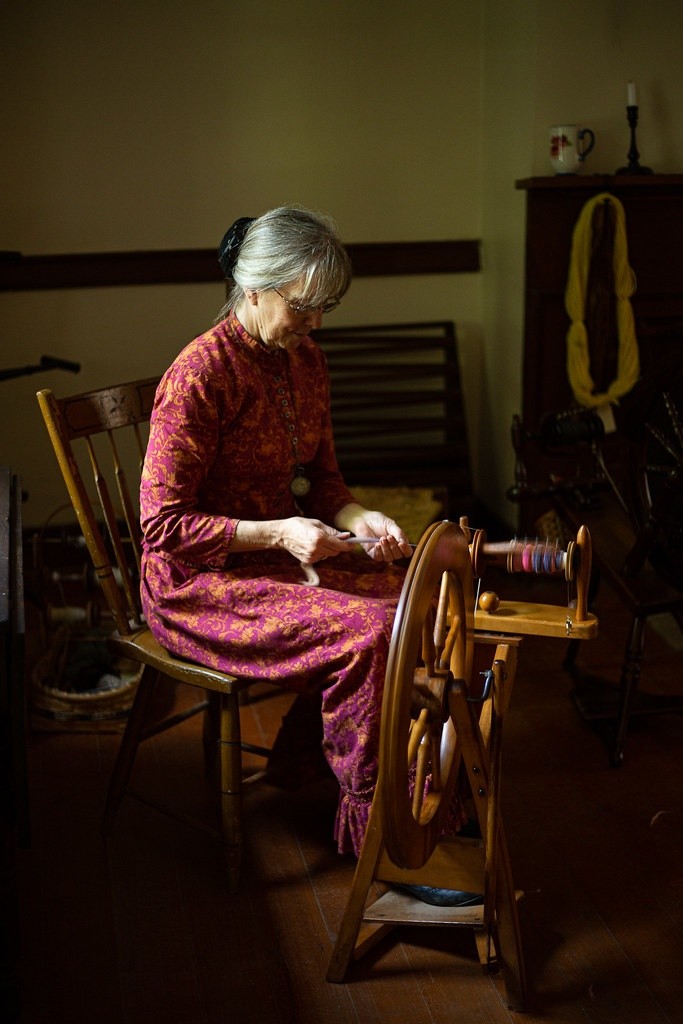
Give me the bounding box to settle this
[27,641,144,734]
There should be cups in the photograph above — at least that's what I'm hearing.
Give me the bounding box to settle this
[547,124,595,175]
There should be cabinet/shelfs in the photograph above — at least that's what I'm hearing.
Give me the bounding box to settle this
[514,170,682,577]
[0,464,29,1024]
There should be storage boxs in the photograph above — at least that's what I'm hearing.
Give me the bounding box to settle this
[29,621,145,718]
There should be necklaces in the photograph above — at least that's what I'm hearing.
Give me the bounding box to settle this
[235,332,310,496]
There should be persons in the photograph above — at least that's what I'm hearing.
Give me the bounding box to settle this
[140,206,485,908]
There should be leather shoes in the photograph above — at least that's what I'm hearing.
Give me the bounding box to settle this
[379,877,483,906]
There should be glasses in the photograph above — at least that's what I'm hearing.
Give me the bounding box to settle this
[271,284,341,314]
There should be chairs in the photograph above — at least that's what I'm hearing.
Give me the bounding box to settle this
[304,322,518,544]
[36,375,332,893]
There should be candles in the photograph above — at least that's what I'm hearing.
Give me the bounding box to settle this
[627,79,637,105]
